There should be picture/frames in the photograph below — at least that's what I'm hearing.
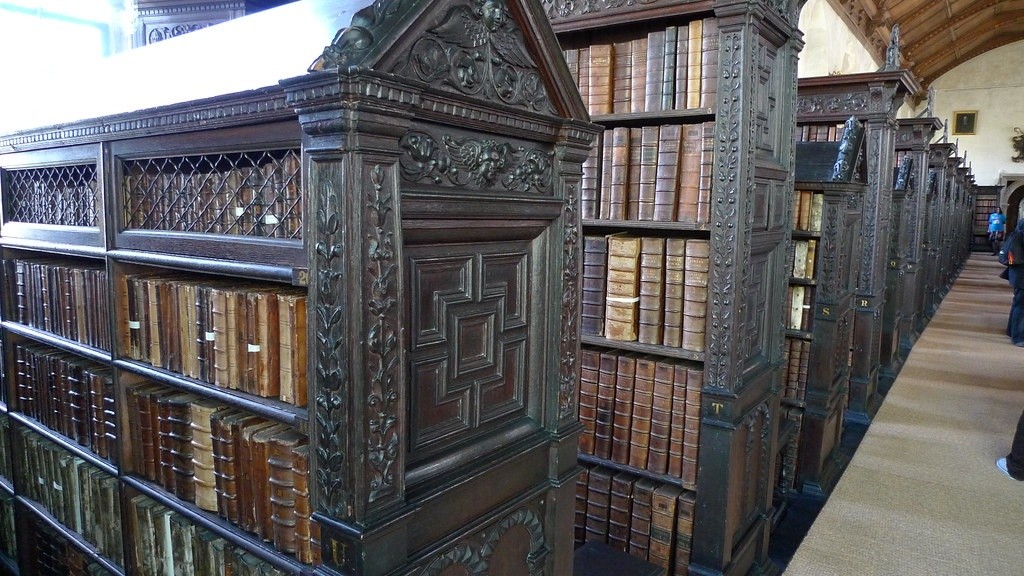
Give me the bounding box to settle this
[952,110,978,135]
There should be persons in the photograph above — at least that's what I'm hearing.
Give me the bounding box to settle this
[987,207,1006,256]
[996,411,1024,481]
[998,219,1024,347]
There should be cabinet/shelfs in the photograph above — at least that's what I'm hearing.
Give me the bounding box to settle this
[0,0,1004,576]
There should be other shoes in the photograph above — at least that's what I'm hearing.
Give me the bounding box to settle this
[991,253,997,256]
[1015,342,1024,347]
[997,458,1016,481]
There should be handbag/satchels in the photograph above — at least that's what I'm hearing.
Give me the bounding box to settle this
[999,266,1009,280]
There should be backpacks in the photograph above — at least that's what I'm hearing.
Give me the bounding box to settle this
[999,229,1024,266]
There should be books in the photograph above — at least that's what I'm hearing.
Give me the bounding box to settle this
[975,194,997,245]
[888,150,914,313]
[929,168,934,174]
[771,116,868,494]
[558,17,719,576]
[0,158,308,576]
